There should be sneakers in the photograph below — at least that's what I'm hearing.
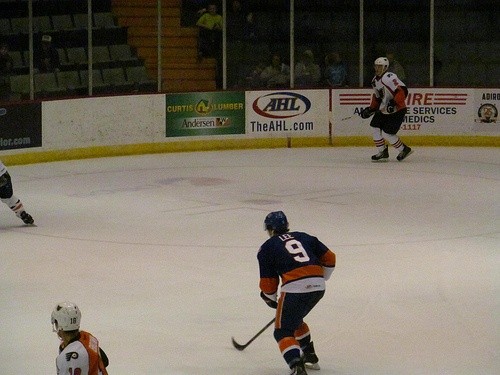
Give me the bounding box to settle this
[394,143,414,162]
[20,210,35,225]
[288,358,309,375]
[371,144,390,163]
[300,341,321,370]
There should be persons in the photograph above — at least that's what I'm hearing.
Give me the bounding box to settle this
[36,38,64,72]
[0,160,35,226]
[255,210,336,375]
[196,0,407,90]
[0,40,17,76]
[50,301,110,375]
[360,55,415,163]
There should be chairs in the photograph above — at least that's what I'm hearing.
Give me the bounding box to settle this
[0,0,164,99]
[182,0,500,88]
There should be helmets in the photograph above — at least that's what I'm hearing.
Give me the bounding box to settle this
[375,57,389,77]
[263,210,288,230]
[50,301,81,331]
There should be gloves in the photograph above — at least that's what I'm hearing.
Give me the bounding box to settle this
[359,107,374,119]
[259,290,278,309]
[385,99,397,114]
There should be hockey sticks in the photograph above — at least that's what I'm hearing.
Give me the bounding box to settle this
[231,315,276,351]
[328,107,387,124]
